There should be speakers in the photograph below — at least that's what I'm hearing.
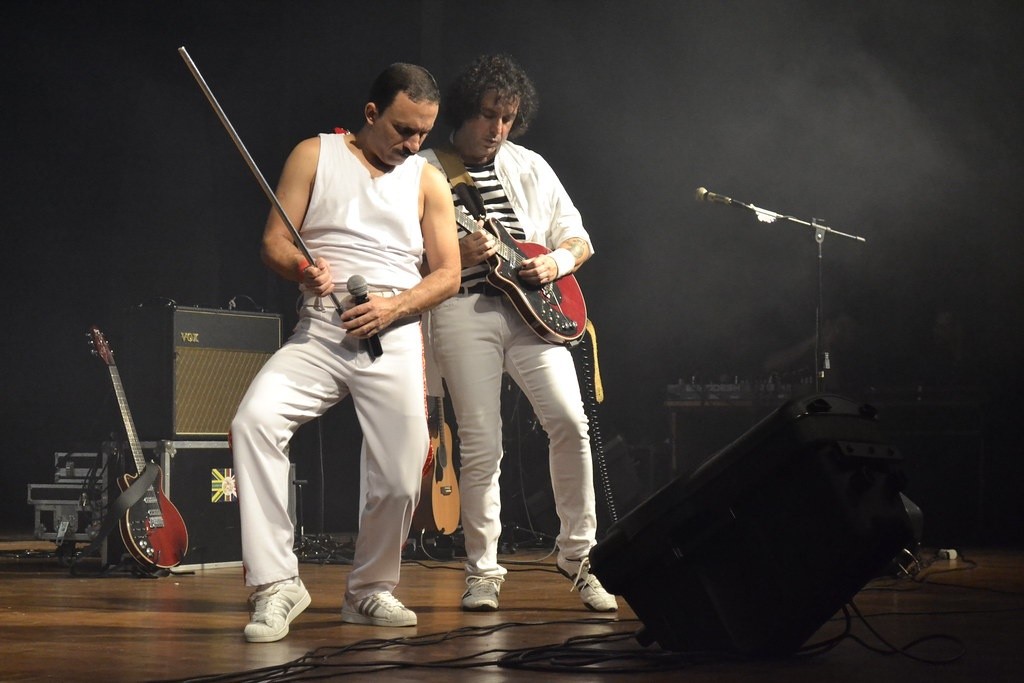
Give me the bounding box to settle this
[162,305,284,442]
[590,392,915,658]
[347,275,383,357]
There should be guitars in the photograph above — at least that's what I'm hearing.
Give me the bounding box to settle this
[85,326,190,571]
[414,397,461,536]
[456,205,588,347]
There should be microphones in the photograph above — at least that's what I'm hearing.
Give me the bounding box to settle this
[694,187,746,208]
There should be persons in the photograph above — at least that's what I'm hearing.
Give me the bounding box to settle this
[228,61,462,641]
[416,55,618,608]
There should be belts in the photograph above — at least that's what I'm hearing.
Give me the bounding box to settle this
[460,282,504,298]
[303,288,404,309]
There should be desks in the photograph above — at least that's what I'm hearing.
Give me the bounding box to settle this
[654,400,767,474]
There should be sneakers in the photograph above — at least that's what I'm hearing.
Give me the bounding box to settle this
[340,590,418,627]
[556,549,618,612]
[460,576,503,612]
[245,576,312,642]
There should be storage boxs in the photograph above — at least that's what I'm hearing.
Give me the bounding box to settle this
[26,439,244,571]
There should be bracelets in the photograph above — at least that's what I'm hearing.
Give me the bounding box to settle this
[546,247,575,281]
[298,259,309,269]
